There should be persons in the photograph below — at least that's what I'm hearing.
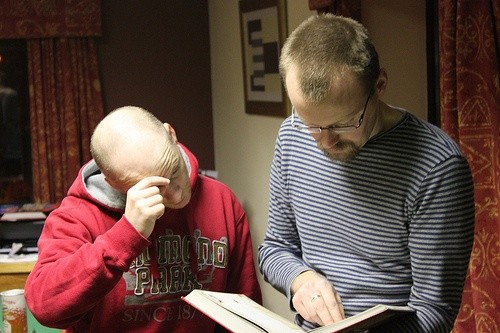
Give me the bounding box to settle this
[258,13,475,333]
[24,106,262,333]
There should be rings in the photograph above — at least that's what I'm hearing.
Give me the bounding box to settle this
[310,293,320,302]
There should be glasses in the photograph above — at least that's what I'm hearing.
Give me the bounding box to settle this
[291,92,370,133]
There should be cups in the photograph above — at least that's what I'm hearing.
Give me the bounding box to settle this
[0,289,28,333]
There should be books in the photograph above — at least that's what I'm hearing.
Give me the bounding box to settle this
[181,289,416,333]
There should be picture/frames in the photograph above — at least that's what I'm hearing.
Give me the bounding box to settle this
[238,0,291,118]
